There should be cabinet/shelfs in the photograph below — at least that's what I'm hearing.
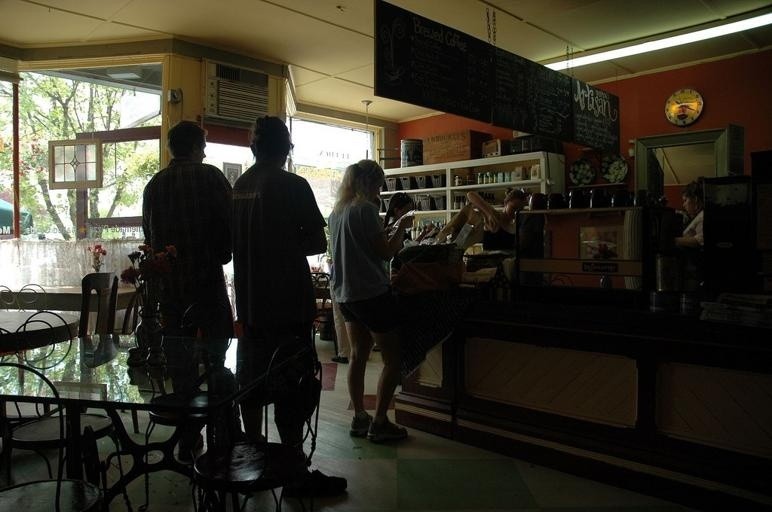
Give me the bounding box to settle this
[451,151,566,215]
[379,162,451,214]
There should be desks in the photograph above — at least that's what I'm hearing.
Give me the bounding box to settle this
[0,308,81,359]
[0,334,308,512]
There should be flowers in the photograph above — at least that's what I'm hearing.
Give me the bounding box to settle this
[90,242,105,272]
[121,245,176,310]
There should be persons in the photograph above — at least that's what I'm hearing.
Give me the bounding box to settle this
[233,117,348,498]
[332,159,414,443]
[467,187,525,252]
[676,175,704,251]
[326,196,384,364]
[142,120,233,462]
[384,193,431,265]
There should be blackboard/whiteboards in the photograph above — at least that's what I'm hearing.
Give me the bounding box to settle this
[492,47,571,144]
[375,0,497,125]
[571,76,620,156]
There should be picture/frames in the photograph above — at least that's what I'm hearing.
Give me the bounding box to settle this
[49,137,102,189]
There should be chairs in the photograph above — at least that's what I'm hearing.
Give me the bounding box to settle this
[78,272,119,336]
[0,361,100,512]
[312,270,339,358]
[194,315,322,512]
[0,311,134,511]
[138,301,239,512]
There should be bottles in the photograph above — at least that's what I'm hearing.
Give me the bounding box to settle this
[454,195,470,209]
[415,221,420,239]
[477,173,498,184]
[411,220,416,240]
[478,192,496,207]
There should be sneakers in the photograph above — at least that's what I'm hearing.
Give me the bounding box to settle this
[332,357,349,363]
[177,433,203,462]
[349,415,373,437]
[283,468,347,496]
[367,422,408,439]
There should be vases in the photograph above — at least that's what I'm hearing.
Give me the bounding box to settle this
[135,306,168,354]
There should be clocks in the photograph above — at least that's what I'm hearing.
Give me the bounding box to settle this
[666,88,704,126]
